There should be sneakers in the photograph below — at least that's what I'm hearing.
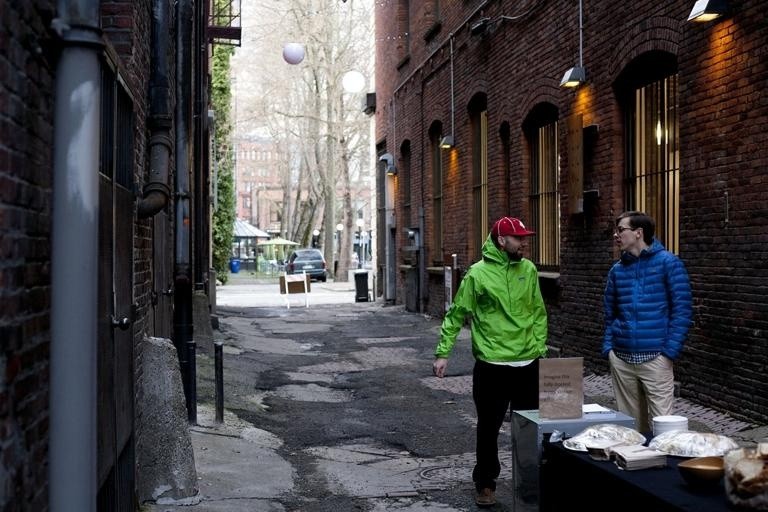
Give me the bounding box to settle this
[474,486,497,506]
[521,495,539,507]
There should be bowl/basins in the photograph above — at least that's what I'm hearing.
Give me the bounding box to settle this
[676,454,726,490]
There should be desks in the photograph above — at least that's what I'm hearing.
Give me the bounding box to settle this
[543,432,729,511]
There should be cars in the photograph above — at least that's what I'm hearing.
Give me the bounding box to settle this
[286,250,326,282]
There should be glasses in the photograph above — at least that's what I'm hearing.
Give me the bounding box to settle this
[614,226,637,233]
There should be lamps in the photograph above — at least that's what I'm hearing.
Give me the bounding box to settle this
[438,39,456,148]
[385,94,398,175]
[560,1,585,87]
[688,1,727,24]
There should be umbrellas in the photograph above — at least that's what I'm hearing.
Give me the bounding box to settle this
[258,235,302,264]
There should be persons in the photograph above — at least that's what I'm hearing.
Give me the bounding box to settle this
[432,217,549,507]
[601,211,693,434]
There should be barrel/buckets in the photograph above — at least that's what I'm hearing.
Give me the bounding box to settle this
[229,259,240,273]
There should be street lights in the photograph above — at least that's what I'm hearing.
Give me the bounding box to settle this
[337,224,343,255]
[356,220,365,268]
[312,230,319,248]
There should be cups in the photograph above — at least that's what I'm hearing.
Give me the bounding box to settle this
[652,415,689,436]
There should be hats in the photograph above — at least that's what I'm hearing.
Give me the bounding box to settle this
[491,216,537,237]
